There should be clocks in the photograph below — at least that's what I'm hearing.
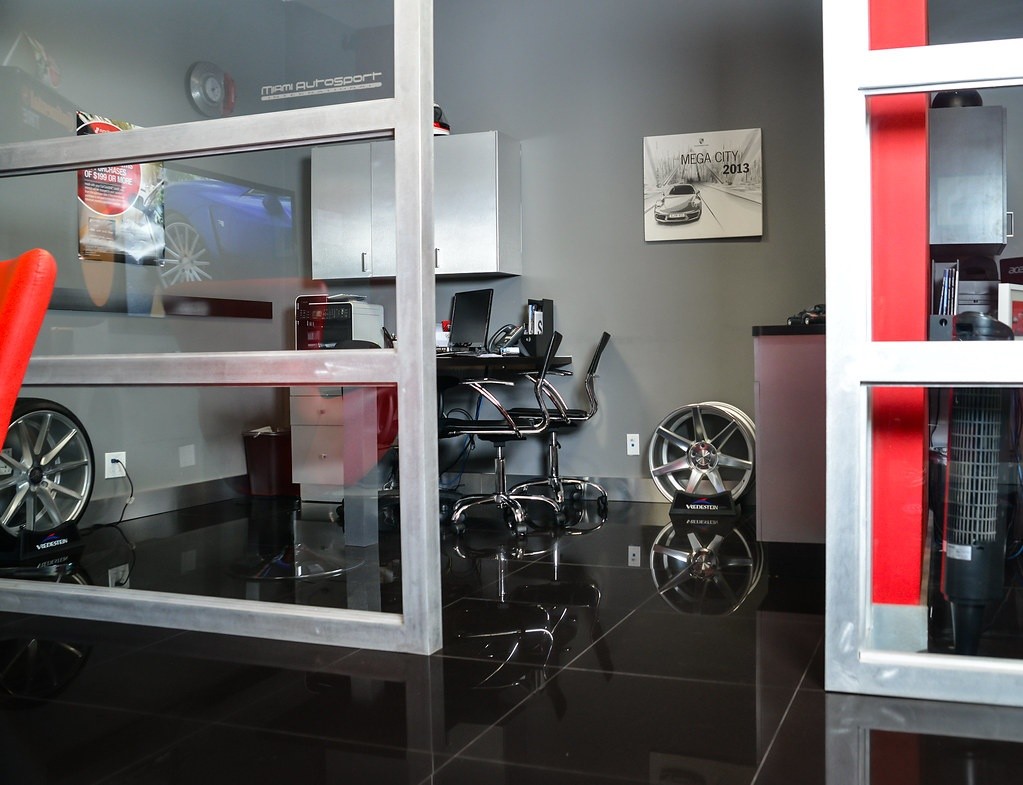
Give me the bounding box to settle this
[189,61,236,118]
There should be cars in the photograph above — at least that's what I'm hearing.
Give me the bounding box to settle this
[655,183,702,224]
[787,303,826,326]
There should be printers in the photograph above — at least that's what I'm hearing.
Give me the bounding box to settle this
[294,294,385,349]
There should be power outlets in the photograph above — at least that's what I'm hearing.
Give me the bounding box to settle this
[0,449,13,475]
[181,549,196,572]
[179,443,195,468]
[105,450,127,481]
[108,564,130,590]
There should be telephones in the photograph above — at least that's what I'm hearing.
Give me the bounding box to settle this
[487,325,524,356]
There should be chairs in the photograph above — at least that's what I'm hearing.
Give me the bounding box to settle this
[455,545,568,724]
[504,532,613,684]
[504,330,612,530]
[438,329,566,544]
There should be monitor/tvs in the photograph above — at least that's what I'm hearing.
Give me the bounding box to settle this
[447,288,494,348]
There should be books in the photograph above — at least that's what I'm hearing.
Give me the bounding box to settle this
[528,303,542,335]
[534,311,544,335]
[937,267,959,327]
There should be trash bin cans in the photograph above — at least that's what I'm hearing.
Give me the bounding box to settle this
[243,426,294,496]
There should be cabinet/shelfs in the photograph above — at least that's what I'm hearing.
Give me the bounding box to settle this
[310,127,523,280]
[289,386,379,503]
[751,324,825,546]
[928,105,1015,257]
